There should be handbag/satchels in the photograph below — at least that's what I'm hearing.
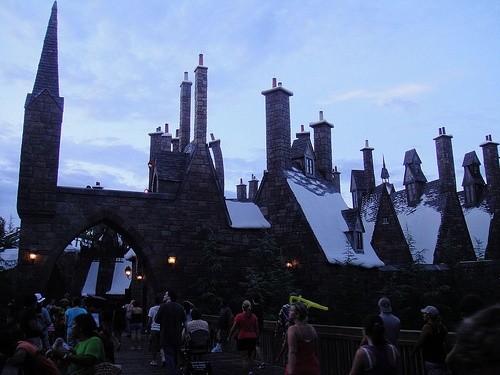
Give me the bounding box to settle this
[91,313,100,327]
[70,340,122,375]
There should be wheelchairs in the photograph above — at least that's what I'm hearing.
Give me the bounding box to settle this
[175,329,213,375]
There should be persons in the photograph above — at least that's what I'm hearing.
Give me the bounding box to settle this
[445,304,500,375]
[144,291,210,375]
[284,303,321,375]
[350,314,401,375]
[16,292,143,375]
[408,306,451,375]
[226,300,265,375]
[359,298,400,348]
[211,298,234,352]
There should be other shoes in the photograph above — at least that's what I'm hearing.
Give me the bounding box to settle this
[210,348,222,352]
[162,358,166,365]
[130,346,134,350]
[258,362,266,368]
[137,346,141,349]
[116,343,121,350]
[150,361,157,365]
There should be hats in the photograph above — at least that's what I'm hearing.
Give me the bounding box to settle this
[35,293,46,303]
[420,306,439,315]
[378,297,392,313]
[60,298,69,303]
